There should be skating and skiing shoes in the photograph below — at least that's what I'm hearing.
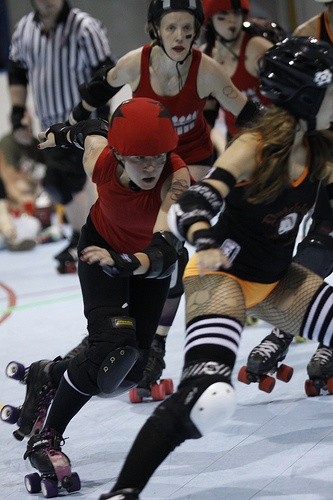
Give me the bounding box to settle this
[24,426,82,498]
[0,355,64,441]
[128,346,174,403]
[237,325,294,394]
[304,343,333,398]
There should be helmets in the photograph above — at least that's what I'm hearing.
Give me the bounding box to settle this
[107,97,179,156]
[146,0,205,40]
[256,37,333,120]
[201,0,250,25]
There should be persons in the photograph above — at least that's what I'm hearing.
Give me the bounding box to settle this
[66,0,263,403]
[0,98,197,498]
[195,0,333,398]
[97,35,333,498]
[0,111,70,250]
[7,0,114,273]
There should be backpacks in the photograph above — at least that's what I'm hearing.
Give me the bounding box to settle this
[241,15,289,45]
